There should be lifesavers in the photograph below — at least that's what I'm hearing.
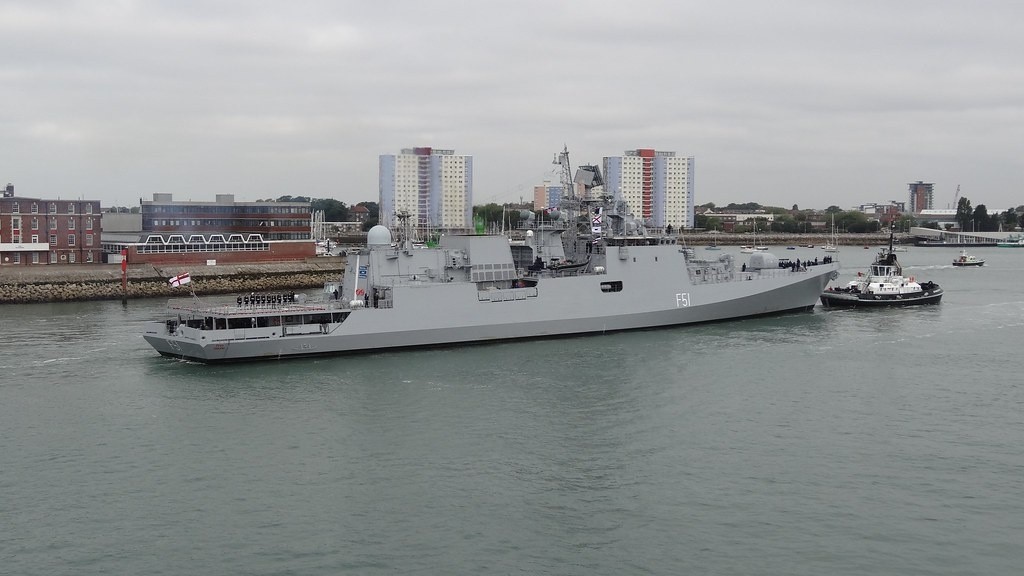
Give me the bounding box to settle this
[360,289,364,296]
[356,289,360,295]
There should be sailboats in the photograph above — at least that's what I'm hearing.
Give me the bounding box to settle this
[823,211,839,251]
[706,226,721,249]
[740,217,768,254]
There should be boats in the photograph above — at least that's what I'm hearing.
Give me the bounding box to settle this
[953,249,986,267]
[142,144,840,364]
[864,245,869,249]
[798,242,814,248]
[997,234,1024,247]
[787,247,796,249]
[820,217,944,308]
[892,244,908,252]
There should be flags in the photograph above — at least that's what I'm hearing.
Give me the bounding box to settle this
[168,271,191,287]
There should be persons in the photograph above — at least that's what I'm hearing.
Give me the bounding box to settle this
[928,281,934,288]
[374,293,379,308]
[364,293,369,308]
[835,285,857,294]
[333,289,338,300]
[585,240,592,253]
[782,255,832,272]
[742,263,746,272]
[668,223,672,234]
[237,289,295,307]
